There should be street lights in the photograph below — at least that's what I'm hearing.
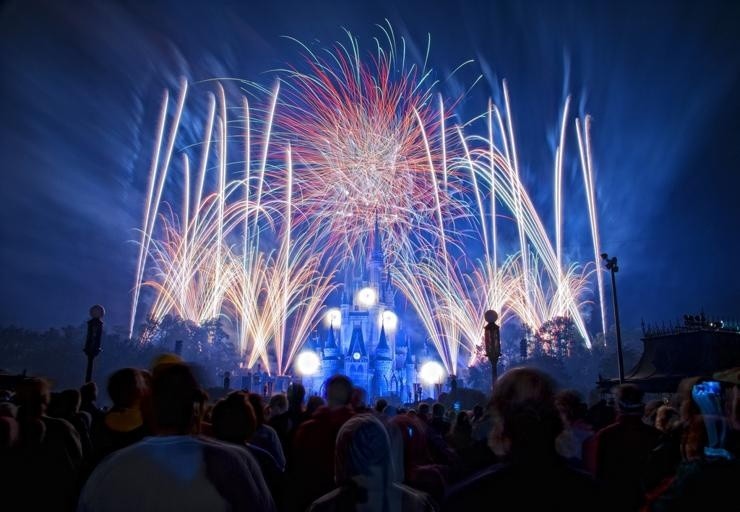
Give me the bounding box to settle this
[418,387,423,401]
[223,371,231,388]
[83,304,103,383]
[480,310,502,386]
[263,383,267,395]
[408,391,412,403]
[314,390,318,395]
[600,251,625,385]
[450,374,457,395]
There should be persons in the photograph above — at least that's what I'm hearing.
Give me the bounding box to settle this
[0,356,739,512]
